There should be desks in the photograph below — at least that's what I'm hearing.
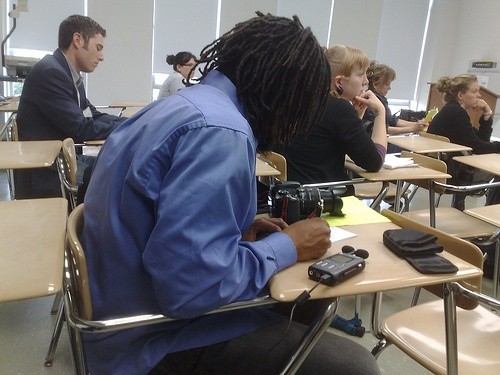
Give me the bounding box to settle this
[0,140,63,169]
[268,220,484,375]
[453,152,500,177]
[384,135,473,163]
[344,161,452,228]
[0,197,68,304]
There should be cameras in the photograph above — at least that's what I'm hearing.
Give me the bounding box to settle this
[307,253,365,286]
[267,181,348,225]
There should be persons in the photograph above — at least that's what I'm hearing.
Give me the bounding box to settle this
[13,14,130,199]
[157,52,198,101]
[80,8,381,375]
[270,43,500,215]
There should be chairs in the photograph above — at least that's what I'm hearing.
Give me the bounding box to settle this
[0,99,500,375]
